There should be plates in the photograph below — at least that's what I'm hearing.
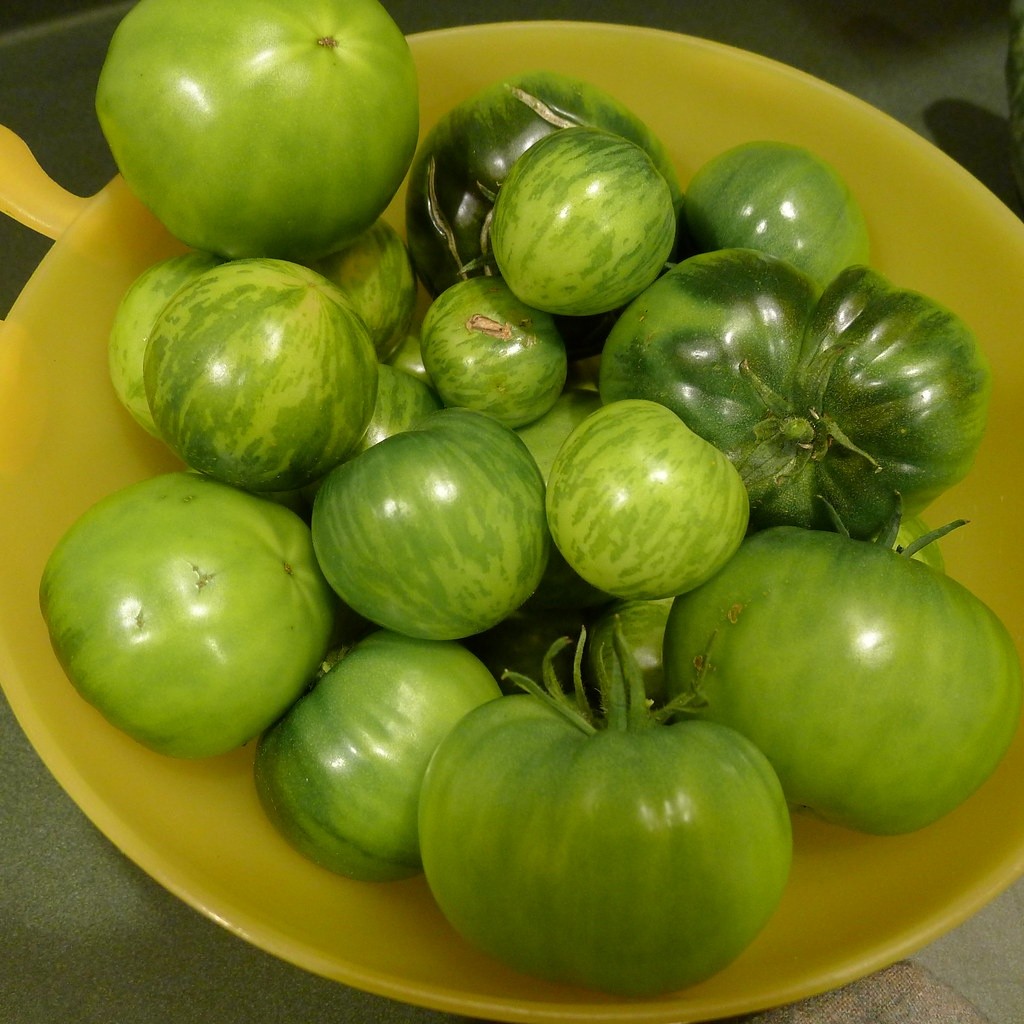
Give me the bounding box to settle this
[0,20,1024,1024]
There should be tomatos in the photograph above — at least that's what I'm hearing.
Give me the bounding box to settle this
[37,1,1021,993]
